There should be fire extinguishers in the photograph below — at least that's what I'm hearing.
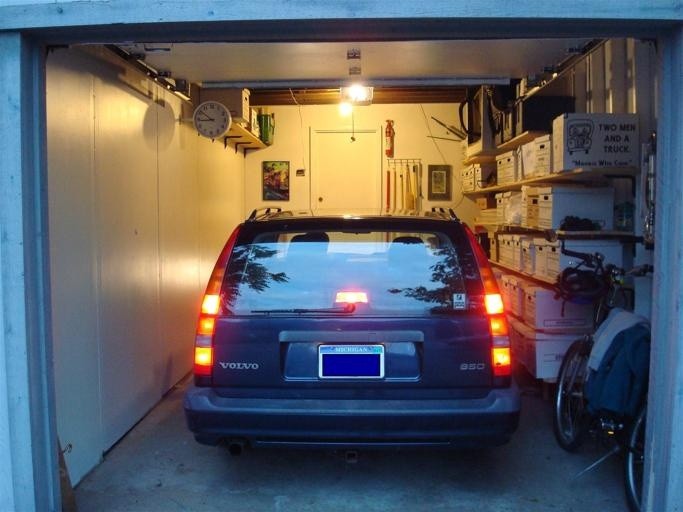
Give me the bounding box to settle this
[384,120,395,158]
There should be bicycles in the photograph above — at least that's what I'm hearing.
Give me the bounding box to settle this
[554,242,654,511]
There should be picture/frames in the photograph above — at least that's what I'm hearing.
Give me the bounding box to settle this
[261,161,290,201]
[428,164,450,200]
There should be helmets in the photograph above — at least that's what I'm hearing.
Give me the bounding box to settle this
[559,268,608,305]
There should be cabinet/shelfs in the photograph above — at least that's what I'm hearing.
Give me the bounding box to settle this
[461,130,644,400]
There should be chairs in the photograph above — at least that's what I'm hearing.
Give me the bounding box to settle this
[289,233,431,267]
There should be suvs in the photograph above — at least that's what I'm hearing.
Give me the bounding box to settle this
[183,204,523,462]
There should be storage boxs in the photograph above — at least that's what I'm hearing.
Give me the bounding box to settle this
[200,87,249,120]
[461,113,640,382]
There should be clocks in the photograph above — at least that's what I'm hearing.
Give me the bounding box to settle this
[193,101,232,139]
